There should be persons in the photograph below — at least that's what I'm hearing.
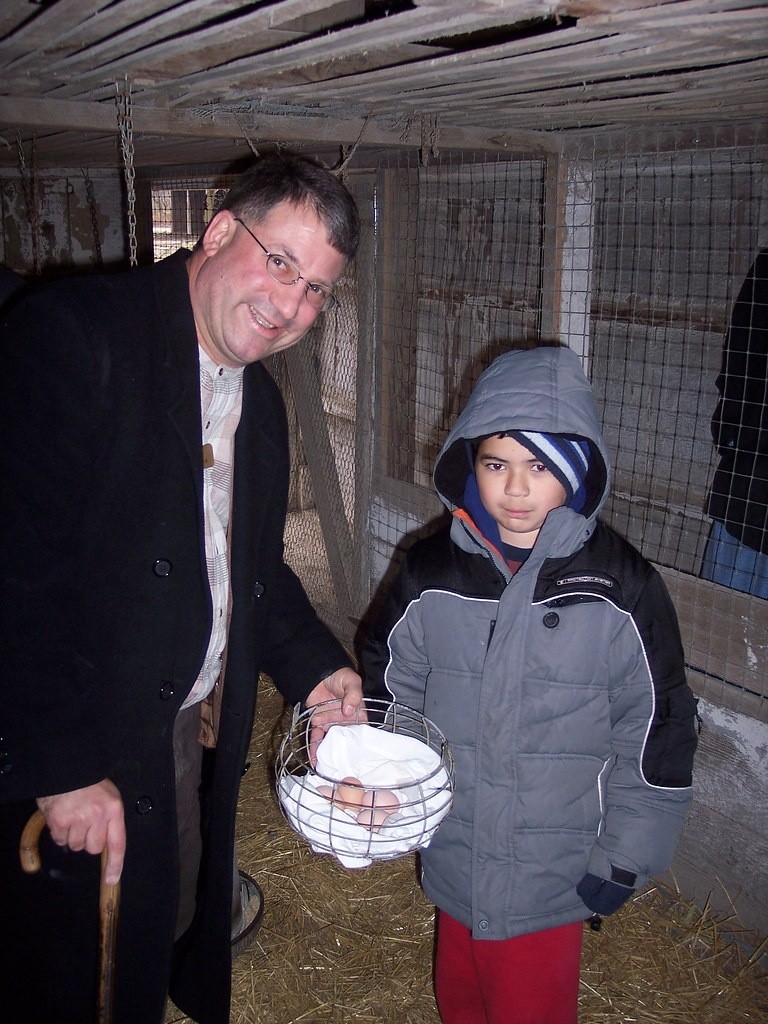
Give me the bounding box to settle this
[352,342,700,1024]
[1,162,368,1024]
[702,245,768,601]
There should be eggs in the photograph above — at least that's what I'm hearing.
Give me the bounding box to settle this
[356,809,389,833]
[315,785,344,811]
[362,789,399,815]
[338,777,365,811]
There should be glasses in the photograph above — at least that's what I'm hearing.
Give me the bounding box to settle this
[234,215,341,313]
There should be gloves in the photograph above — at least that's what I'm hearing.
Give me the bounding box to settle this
[575,875,637,917]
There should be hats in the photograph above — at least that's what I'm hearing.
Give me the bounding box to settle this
[507,431,596,506]
